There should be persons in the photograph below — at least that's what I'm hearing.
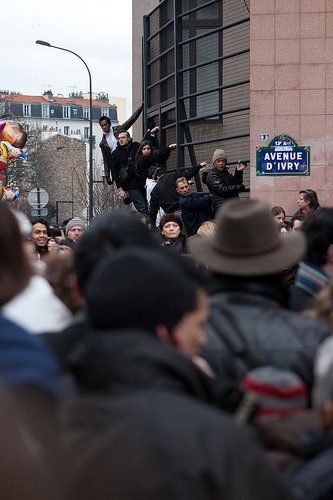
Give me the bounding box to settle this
[109,122,178,215]
[0,190,333,500]
[205,149,249,200]
[175,176,215,236]
[144,162,206,227]
[98,103,143,184]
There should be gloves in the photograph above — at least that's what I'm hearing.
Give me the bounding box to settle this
[106,178,113,186]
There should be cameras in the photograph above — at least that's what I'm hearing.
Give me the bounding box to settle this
[47,228,65,236]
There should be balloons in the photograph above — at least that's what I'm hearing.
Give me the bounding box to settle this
[0,120,27,201]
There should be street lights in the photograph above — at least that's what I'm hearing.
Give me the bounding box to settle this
[55,201,75,228]
[71,158,95,218]
[35,39,94,225]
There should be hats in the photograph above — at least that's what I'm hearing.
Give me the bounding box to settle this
[212,148,228,165]
[64,217,86,235]
[182,197,306,277]
[159,214,181,231]
